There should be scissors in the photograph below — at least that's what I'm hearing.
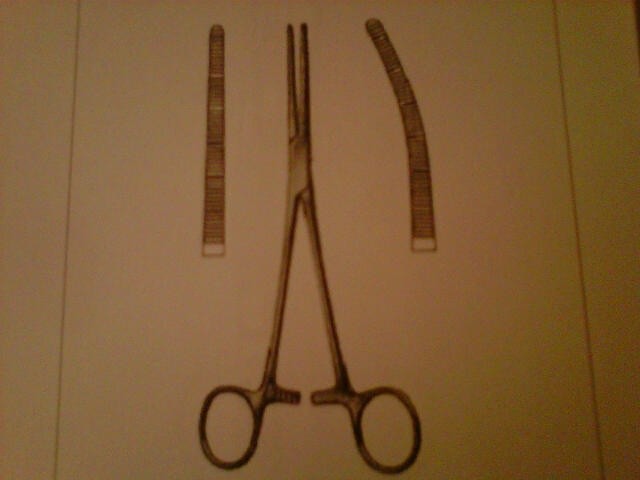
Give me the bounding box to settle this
[198,22,422,474]
